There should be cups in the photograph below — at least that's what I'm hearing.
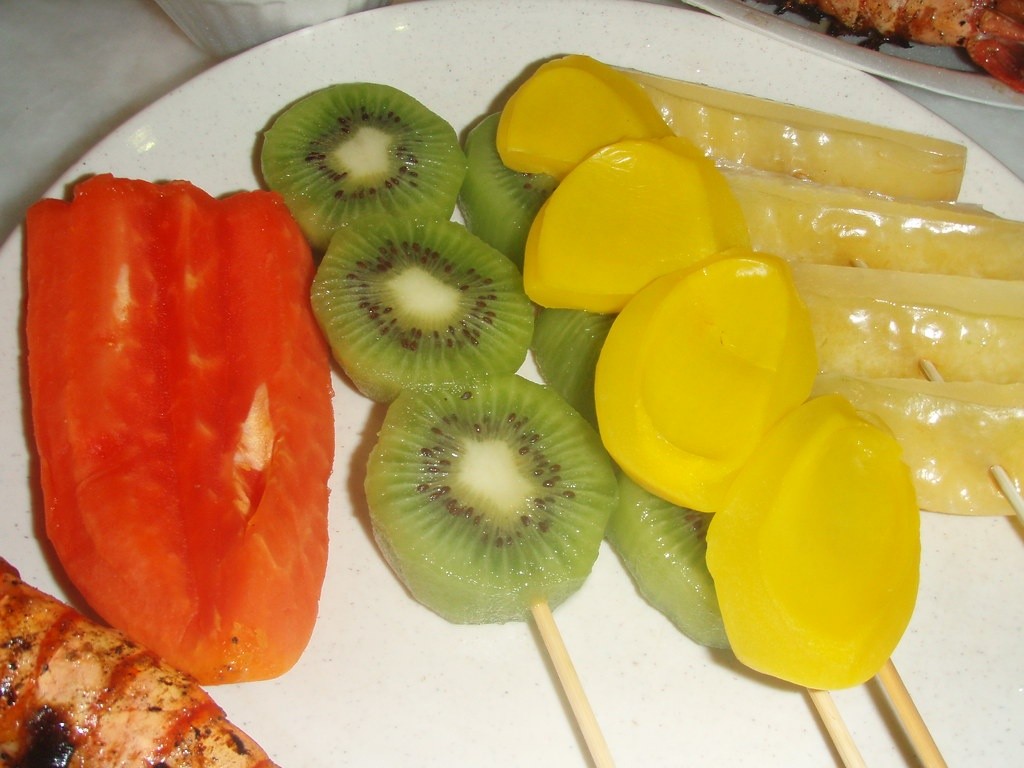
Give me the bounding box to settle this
[155,0,387,58]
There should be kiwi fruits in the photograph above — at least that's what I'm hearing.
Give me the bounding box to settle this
[258,81,736,651]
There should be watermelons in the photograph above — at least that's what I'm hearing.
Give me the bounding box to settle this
[19,172,334,682]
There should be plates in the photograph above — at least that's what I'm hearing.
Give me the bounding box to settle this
[683,0,1024,113]
[0,0,1024,768]
